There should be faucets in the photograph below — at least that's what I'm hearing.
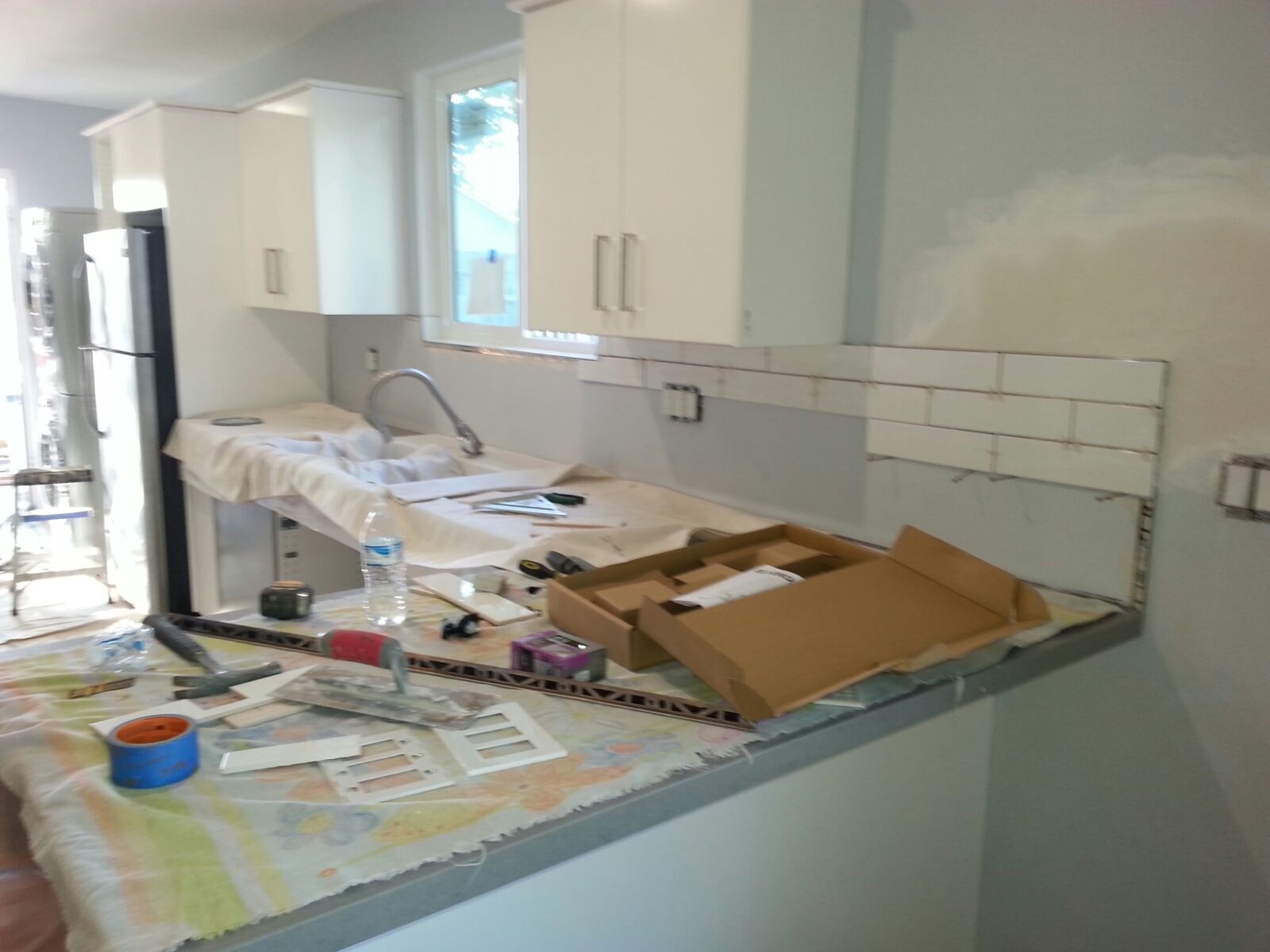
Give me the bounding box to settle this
[360,368,486,456]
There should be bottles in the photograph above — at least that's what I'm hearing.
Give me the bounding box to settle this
[357,485,408,628]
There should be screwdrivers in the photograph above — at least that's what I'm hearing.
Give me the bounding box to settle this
[547,551,584,575]
[520,559,566,580]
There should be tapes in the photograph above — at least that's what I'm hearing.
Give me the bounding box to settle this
[107,713,200,790]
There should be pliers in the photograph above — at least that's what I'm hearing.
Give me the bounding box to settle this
[536,491,584,504]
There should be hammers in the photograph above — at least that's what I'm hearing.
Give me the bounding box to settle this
[144,614,282,699]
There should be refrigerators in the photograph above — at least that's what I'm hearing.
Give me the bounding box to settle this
[79,220,214,621]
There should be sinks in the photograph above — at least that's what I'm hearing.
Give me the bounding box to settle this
[280,439,503,490]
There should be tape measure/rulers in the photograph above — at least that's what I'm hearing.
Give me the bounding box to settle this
[262,581,316,621]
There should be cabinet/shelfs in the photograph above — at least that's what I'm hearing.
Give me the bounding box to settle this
[506,2,852,355]
[221,75,420,318]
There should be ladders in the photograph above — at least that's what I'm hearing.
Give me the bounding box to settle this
[11,466,113,615]
